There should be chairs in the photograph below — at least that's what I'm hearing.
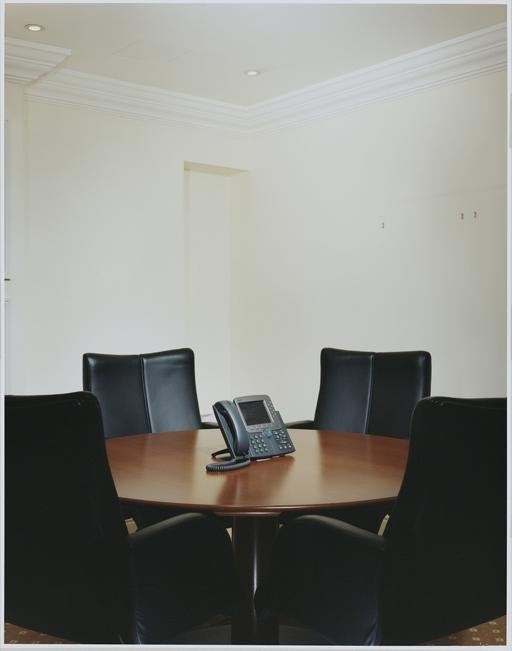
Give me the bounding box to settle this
[270,397,507,645]
[84,349,235,530]
[6,392,232,644]
[285,349,432,533]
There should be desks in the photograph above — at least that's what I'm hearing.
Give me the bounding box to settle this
[106,428,408,645]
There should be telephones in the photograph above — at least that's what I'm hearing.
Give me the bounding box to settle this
[212,395,296,463]
